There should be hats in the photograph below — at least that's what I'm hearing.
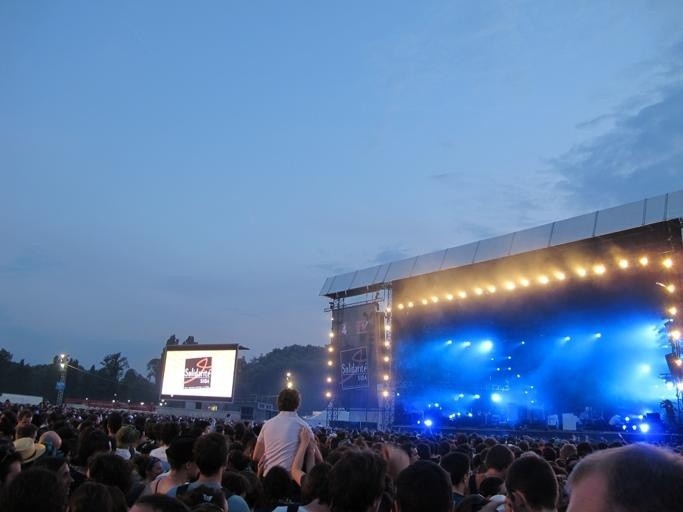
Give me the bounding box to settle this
[12,437,46,464]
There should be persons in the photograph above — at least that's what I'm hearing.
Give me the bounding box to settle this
[0,399,682,512]
[252,388,323,480]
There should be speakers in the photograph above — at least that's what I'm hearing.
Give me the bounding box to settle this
[665,352,677,373]
[647,413,661,431]
[664,321,671,329]
